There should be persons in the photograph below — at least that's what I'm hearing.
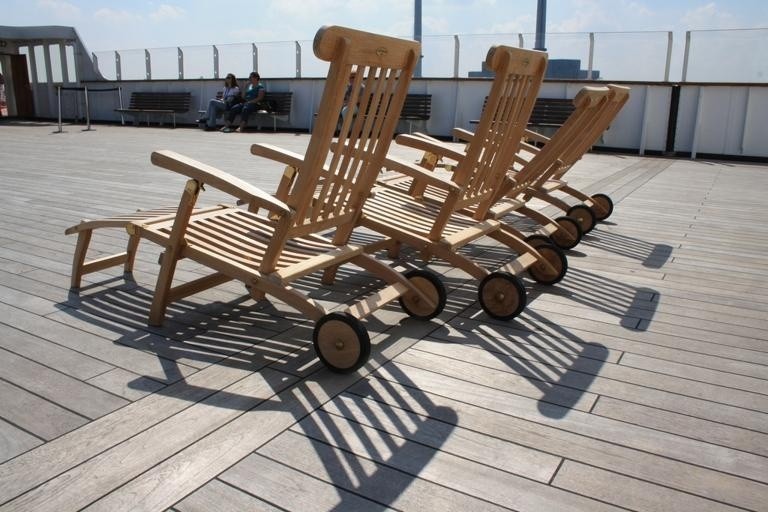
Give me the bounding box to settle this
[340,72,365,120]
[218,72,266,132]
[195,73,243,131]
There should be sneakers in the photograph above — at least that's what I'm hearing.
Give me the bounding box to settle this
[195,118,245,133]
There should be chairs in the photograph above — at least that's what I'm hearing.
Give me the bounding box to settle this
[63,24,446,375]
[237,42,568,322]
[410,84,632,255]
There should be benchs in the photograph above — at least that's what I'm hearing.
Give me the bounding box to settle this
[113,90,192,128]
[312,90,432,130]
[198,90,293,132]
